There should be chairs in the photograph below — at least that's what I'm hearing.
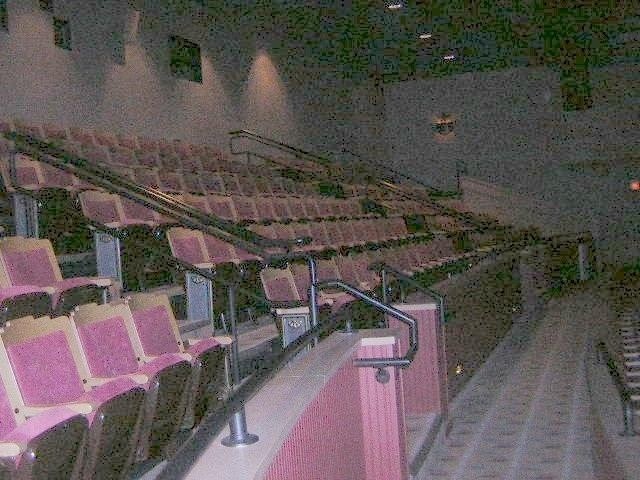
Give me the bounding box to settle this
[75,189,378,235]
[1,314,147,480]
[127,291,234,433]
[165,218,416,286]
[68,303,191,464]
[0,334,88,480]
[2,236,114,314]
[1,122,320,198]
[259,236,476,321]
[1,236,51,318]
